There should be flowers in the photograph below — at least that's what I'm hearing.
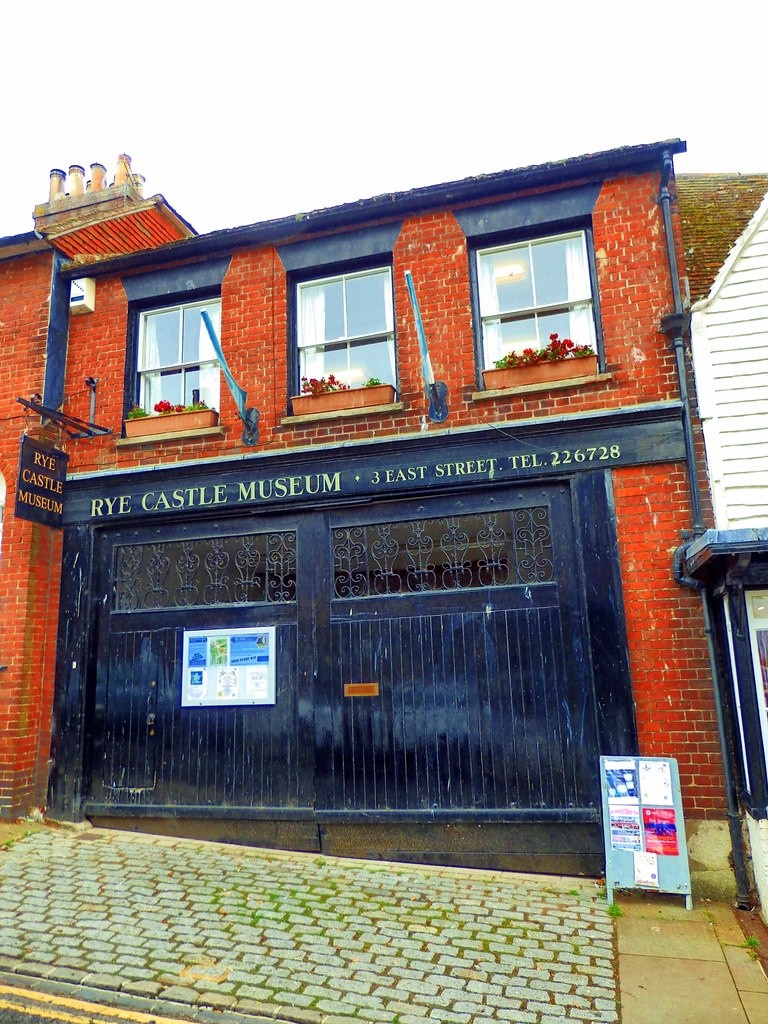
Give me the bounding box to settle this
[298,374,387,397]
[492,332,597,372]
[124,398,217,420]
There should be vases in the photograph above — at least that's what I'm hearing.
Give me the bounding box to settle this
[124,410,218,438]
[479,353,601,391]
[287,383,396,417]
